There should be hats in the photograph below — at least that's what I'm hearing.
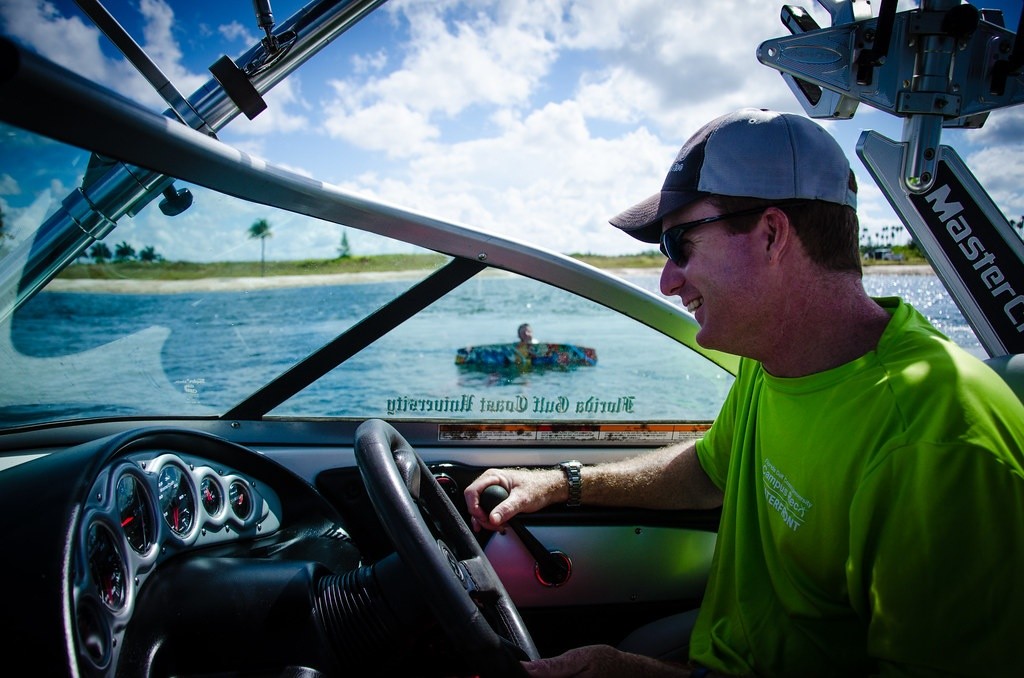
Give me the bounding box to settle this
[608,107,857,244]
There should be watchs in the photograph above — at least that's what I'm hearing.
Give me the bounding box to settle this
[558,460,583,509]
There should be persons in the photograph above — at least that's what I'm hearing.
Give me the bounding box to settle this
[517,323,537,344]
[463,110,1023,677]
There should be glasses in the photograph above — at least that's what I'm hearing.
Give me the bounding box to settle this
[659,205,796,267]
